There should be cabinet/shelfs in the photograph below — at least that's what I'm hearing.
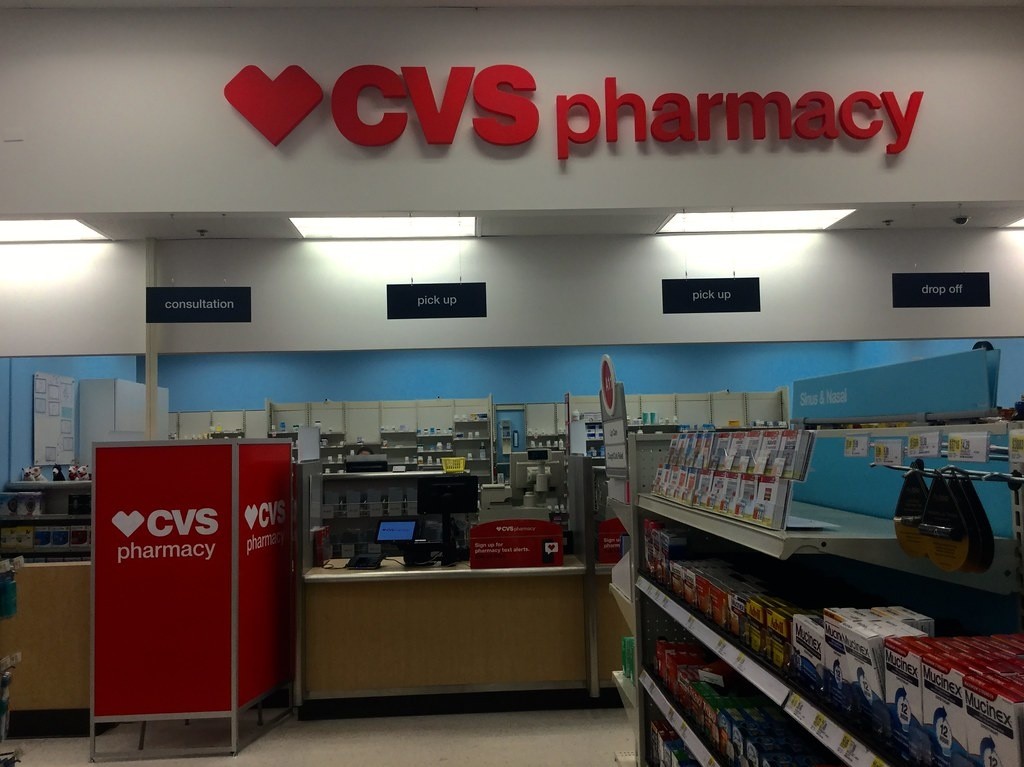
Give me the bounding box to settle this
[605,423,1024,767]
[208,414,605,484]
[79,378,169,477]
[0,480,92,555]
[595,561,619,707]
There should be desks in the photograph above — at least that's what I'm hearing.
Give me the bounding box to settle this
[0,560,118,740]
[296,554,589,722]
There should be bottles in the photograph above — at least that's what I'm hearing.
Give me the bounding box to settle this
[271,412,679,479]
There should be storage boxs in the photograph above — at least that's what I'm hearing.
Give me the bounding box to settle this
[644,430,1024,765]
